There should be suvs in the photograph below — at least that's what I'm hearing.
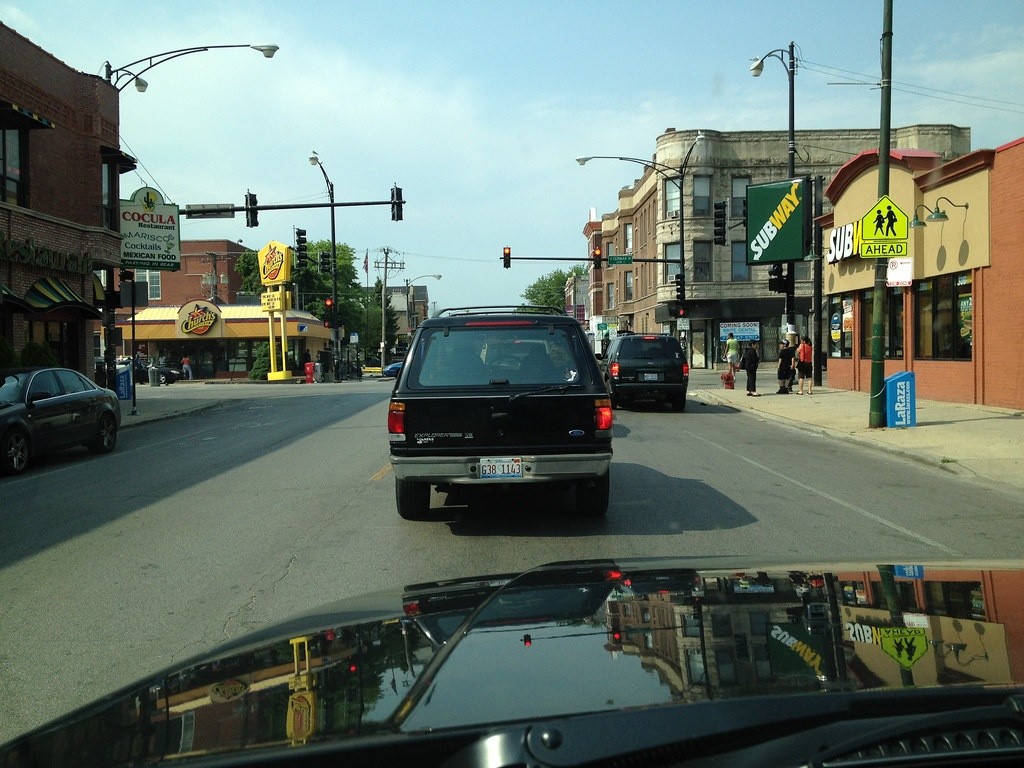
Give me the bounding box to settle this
[503,339,548,355]
[622,568,702,596]
[401,559,623,652]
[388,305,689,520]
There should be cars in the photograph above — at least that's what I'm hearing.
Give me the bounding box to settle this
[0,368,121,475]
[383,362,404,377]
[116,359,178,385]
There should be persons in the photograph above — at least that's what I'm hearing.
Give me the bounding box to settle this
[284,348,320,376]
[181,354,194,379]
[355,353,365,382]
[743,339,761,396]
[777,336,814,397]
[724,333,739,376]
[158,353,166,365]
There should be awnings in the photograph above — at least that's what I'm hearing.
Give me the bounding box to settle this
[0,271,106,323]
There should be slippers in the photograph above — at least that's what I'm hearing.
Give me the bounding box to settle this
[796,391,803,394]
[807,392,812,395]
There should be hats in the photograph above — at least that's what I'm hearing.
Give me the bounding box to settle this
[777,339,789,346]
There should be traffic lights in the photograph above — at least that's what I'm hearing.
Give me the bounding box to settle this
[612,627,621,644]
[349,658,357,672]
[676,275,688,317]
[503,247,511,268]
[594,248,601,268]
[296,229,330,273]
[524,635,531,647]
[714,201,726,246]
[324,298,332,327]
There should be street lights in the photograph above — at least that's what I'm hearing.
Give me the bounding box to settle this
[104,43,279,390]
[406,274,442,331]
[575,135,707,354]
[308,156,341,383]
[750,40,795,347]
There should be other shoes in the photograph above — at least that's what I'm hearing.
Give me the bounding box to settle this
[746,393,753,396]
[753,394,761,396]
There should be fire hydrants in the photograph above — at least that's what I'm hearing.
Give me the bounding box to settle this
[721,372,736,389]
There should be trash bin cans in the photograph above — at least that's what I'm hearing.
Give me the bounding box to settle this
[884,372,916,428]
[115,369,132,400]
[148,370,160,387]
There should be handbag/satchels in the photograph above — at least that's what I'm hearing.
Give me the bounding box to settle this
[796,359,806,369]
[739,351,746,370]
